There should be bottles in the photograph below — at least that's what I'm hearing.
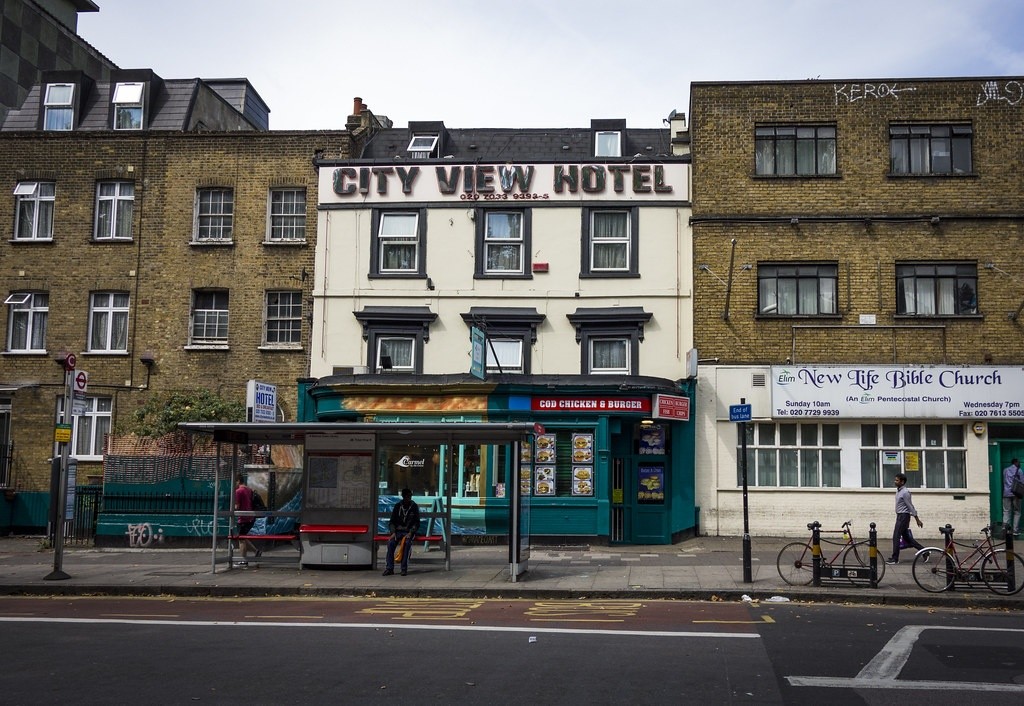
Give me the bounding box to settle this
[844,530,848,539]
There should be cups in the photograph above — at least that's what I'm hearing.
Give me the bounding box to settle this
[425,492,429,496]
[398,491,402,496]
[470,474,480,490]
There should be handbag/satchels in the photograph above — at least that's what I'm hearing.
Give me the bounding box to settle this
[394,536,406,562]
[899,529,913,549]
[1011,468,1024,498]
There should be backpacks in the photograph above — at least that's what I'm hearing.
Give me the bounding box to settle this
[246,487,267,519]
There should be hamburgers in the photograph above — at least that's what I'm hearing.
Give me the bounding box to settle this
[538,482,549,493]
[537,438,548,448]
[575,438,587,448]
[538,451,548,461]
[574,451,584,462]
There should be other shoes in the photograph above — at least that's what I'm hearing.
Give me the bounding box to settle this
[255,550,262,562]
[1013,530,1023,535]
[382,569,394,576]
[232,560,248,568]
[401,570,406,576]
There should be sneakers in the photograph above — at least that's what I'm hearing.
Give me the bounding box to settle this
[923,551,931,563]
[886,558,900,565]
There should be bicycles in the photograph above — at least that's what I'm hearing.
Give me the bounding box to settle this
[912,521,1024,596]
[776,519,885,586]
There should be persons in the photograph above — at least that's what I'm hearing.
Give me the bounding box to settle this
[235,474,262,568]
[886,474,931,565]
[1002,459,1024,535]
[382,488,420,576]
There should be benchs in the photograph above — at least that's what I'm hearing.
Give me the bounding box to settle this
[300,525,376,572]
[228,532,300,570]
[375,536,449,570]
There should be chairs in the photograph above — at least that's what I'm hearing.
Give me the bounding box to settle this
[380,356,399,376]
